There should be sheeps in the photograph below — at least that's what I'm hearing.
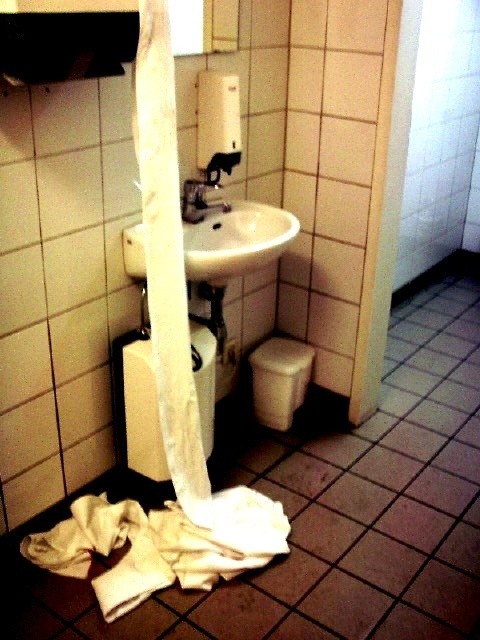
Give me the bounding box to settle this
[184,178,230,222]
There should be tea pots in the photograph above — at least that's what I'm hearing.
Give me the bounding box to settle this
[167,0,240,56]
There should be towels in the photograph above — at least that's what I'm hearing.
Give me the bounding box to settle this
[250,336,316,432]
